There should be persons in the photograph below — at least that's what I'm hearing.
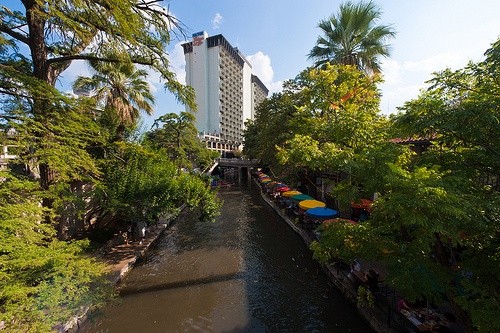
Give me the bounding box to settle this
[398,298,411,314]
[269,192,321,242]
[349,259,361,273]
[366,269,379,293]
[440,312,466,333]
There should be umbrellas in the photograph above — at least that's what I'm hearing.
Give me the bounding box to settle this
[322,218,357,227]
[299,199,326,210]
[306,206,338,218]
[254,169,313,201]
